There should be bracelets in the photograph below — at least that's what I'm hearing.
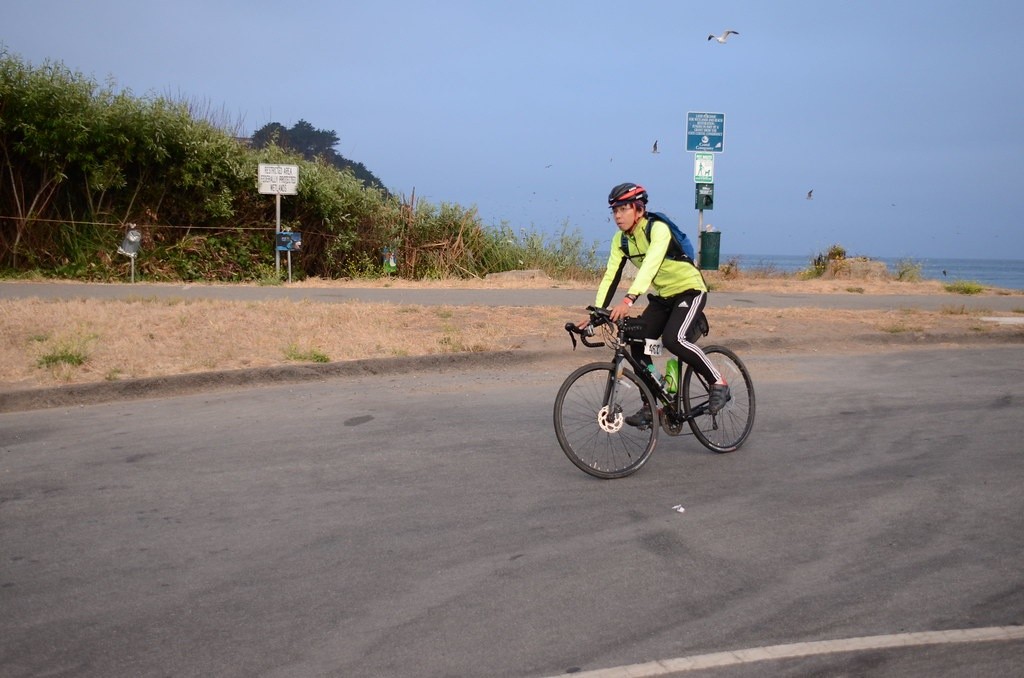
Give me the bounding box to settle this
[623,294,635,306]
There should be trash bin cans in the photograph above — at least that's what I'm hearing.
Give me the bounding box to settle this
[701,231,722,270]
[117,231,141,259]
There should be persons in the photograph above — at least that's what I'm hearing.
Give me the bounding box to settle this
[575,182,730,425]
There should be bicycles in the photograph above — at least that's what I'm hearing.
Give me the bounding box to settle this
[551,303,754,477]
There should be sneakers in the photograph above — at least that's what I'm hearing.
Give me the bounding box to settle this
[708,382,732,412]
[624,406,658,426]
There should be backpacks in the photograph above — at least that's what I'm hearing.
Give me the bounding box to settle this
[645,211,694,262]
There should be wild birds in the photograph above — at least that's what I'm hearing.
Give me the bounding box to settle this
[707,29,740,45]
[804,189,814,201]
[650,140,660,154]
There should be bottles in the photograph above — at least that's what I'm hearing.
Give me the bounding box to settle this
[667,355,678,394]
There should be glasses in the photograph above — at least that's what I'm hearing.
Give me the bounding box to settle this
[610,206,634,214]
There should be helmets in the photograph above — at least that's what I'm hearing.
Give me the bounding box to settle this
[608,183,649,204]
